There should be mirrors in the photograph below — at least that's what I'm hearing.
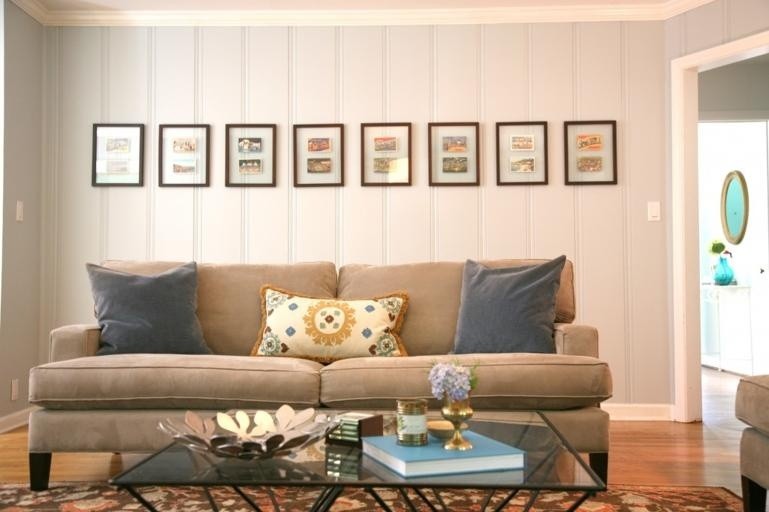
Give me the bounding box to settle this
[719,169,750,245]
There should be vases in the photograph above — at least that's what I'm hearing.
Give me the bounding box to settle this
[442,394,473,451]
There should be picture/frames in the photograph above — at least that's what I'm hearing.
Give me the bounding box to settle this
[360,122,412,187]
[427,121,480,187]
[91,122,145,187]
[563,120,618,186]
[292,123,346,187]
[224,123,277,188]
[158,123,211,188]
[495,121,548,186]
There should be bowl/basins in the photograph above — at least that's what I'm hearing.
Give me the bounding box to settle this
[425,420,470,442]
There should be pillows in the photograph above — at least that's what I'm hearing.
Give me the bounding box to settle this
[245,283,411,364]
[337,258,576,357]
[83,261,214,357]
[100,258,339,358]
[445,252,571,355]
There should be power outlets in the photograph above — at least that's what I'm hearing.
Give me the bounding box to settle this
[16,199,24,221]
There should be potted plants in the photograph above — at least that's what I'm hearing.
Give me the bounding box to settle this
[712,243,734,286]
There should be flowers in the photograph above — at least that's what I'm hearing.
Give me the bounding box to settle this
[421,359,483,402]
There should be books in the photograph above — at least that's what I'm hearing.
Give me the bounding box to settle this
[359,454,525,488]
[357,426,528,478]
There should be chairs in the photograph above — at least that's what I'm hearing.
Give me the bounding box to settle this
[734,373,769,512]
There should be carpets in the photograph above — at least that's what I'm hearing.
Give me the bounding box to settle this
[0,480,744,512]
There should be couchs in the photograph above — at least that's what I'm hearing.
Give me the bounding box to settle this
[26,257,613,491]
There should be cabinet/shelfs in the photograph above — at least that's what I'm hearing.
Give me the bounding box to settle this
[699,281,755,376]
